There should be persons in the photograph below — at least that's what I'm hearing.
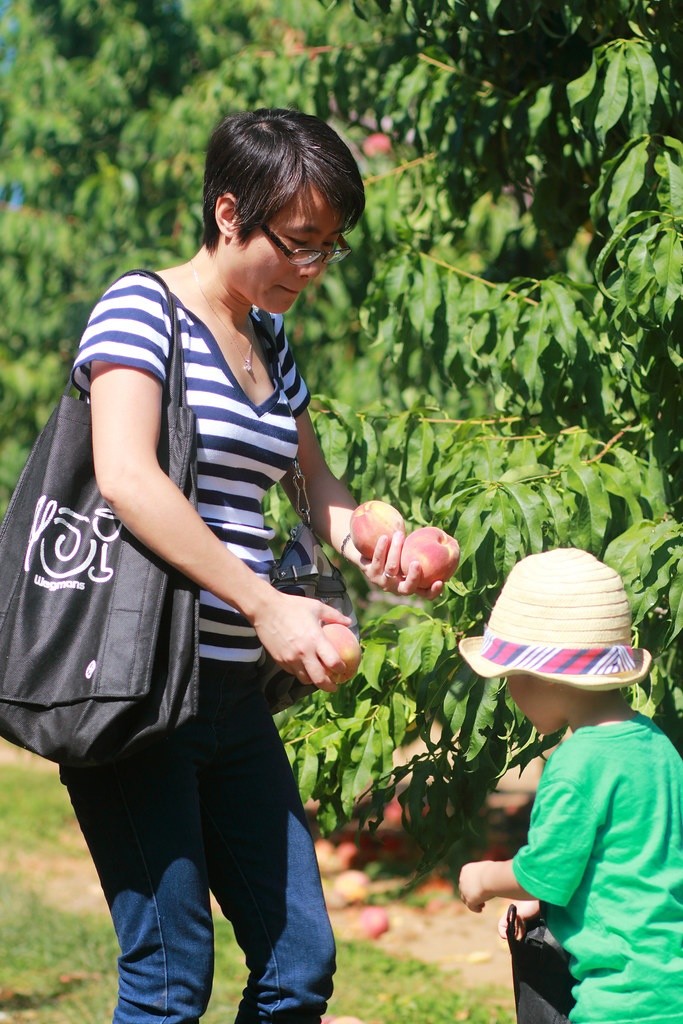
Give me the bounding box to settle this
[62,107,444,1023]
[457,549,683,1024]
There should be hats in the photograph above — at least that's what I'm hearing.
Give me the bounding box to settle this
[456,548,652,692]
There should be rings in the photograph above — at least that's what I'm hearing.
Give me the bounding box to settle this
[384,573,396,580]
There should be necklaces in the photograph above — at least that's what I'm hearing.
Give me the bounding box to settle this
[191,261,253,372]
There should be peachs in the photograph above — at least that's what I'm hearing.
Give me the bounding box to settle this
[400,527,461,589]
[320,1014,366,1024]
[361,131,393,158]
[304,790,501,940]
[349,500,406,560]
[317,622,361,684]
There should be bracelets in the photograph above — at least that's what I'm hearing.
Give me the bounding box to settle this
[341,533,351,561]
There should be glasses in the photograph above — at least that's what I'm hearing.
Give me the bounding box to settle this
[261,222,352,271]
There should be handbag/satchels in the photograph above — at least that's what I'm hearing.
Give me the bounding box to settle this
[250,522,354,713]
[0,268,198,762]
[503,905,575,1024]
